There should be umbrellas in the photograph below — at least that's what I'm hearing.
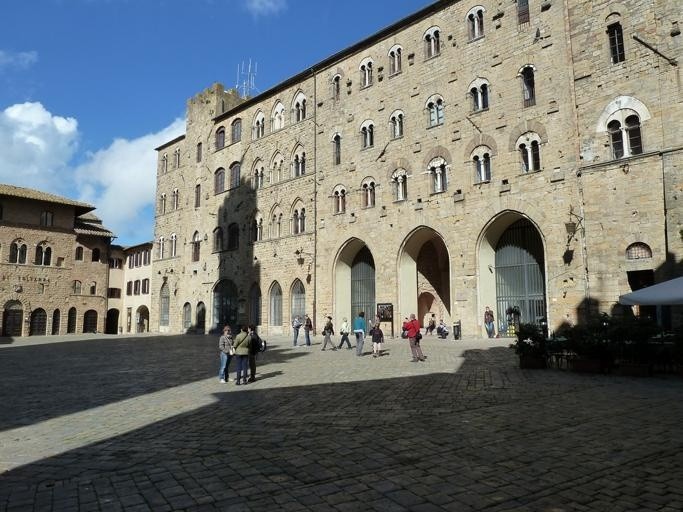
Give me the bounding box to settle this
[617,275,683,309]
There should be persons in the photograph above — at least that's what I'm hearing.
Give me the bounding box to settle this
[401,314,425,362]
[352,311,366,357]
[247,324,259,383]
[452,313,460,341]
[320,316,337,352]
[216,324,233,382]
[230,325,251,385]
[424,313,436,335]
[439,325,448,339]
[337,317,352,349]
[436,318,445,333]
[367,323,383,357]
[367,315,381,353]
[303,313,312,346]
[483,307,495,337]
[291,315,302,347]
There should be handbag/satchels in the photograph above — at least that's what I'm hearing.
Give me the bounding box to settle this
[230,348,235,356]
[416,333,421,339]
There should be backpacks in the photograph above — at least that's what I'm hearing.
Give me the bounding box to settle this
[252,333,265,352]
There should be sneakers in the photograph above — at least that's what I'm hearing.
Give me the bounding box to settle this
[220,376,253,384]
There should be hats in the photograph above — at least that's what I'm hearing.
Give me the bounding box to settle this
[223,326,231,331]
